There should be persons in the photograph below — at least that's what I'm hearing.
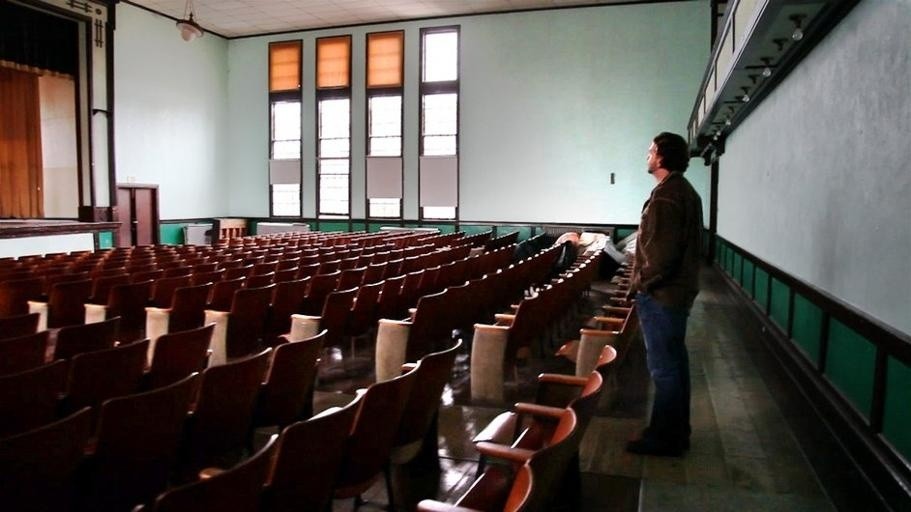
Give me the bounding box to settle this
[626,130,703,457]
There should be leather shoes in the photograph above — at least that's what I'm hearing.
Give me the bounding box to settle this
[623,441,690,457]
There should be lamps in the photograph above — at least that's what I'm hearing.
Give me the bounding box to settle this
[176,0,205,42]
[701,11,806,162]
